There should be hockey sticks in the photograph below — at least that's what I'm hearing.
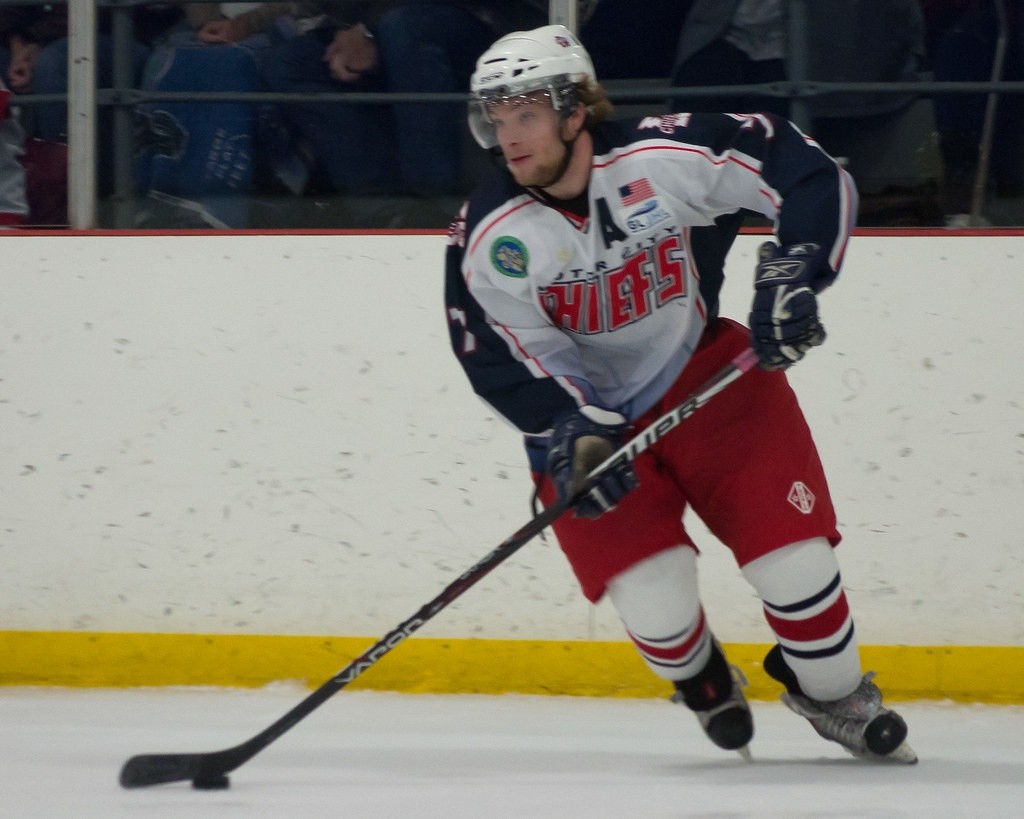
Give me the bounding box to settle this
[118,347,761,794]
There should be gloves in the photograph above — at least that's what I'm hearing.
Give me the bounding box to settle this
[749,241,827,372]
[548,405,638,521]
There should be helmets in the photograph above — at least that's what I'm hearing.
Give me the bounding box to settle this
[470,24,597,123]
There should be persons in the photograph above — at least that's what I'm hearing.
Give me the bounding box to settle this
[0,0,949,227]
[444,25,907,755]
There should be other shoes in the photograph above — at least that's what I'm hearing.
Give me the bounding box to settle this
[151,169,251,230]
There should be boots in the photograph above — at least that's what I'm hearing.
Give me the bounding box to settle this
[764,641,918,766]
[669,628,756,766]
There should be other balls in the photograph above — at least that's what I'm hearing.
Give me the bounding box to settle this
[194,774,229,790]
